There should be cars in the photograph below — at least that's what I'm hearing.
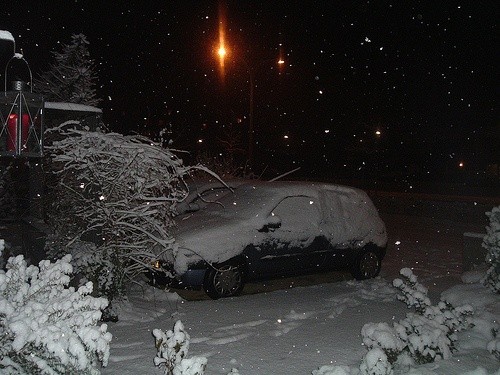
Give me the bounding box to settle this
[139,181,390,300]
[162,177,254,217]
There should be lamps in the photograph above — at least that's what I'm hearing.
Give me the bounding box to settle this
[0,53,45,159]
